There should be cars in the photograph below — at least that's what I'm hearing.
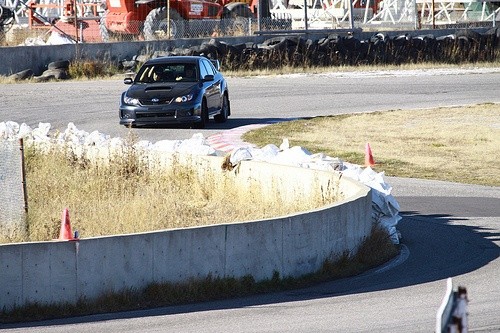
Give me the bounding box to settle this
[118,55,230,128]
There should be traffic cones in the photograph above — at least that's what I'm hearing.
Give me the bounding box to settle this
[361,144,378,168]
[53,209,80,243]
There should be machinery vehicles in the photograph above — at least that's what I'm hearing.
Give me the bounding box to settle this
[0,0,433,42]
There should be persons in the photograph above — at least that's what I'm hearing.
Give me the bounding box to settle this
[183,64,195,78]
